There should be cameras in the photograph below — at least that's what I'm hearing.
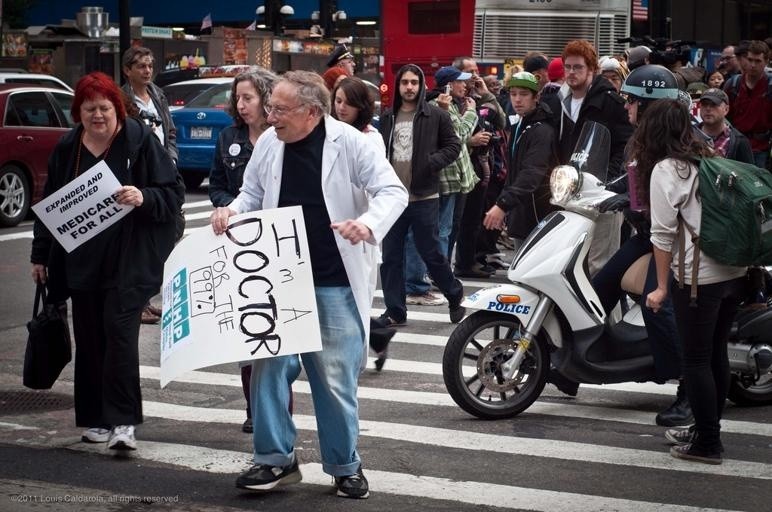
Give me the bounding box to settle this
[649,40,691,66]
[617,35,656,48]
[485,128,504,147]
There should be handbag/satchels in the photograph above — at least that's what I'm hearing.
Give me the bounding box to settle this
[23,301,72,390]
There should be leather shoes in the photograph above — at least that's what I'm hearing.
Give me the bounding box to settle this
[141,309,160,324]
[375,350,386,371]
[148,306,163,316]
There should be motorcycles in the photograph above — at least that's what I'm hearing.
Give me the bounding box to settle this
[441,122,767,417]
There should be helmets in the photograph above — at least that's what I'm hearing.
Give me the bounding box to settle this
[620,64,679,100]
[508,71,538,92]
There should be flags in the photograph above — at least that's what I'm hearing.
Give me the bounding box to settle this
[632,0,650,21]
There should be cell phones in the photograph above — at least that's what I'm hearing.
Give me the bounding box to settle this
[444,84,450,96]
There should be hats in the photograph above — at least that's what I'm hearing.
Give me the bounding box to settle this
[600,58,621,75]
[548,58,564,80]
[327,43,354,68]
[435,67,472,87]
[698,88,729,105]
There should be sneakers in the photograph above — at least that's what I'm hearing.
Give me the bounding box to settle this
[424,276,442,291]
[82,428,111,442]
[108,425,137,451]
[546,369,578,396]
[449,286,465,323]
[670,445,723,464]
[377,313,405,326]
[236,455,303,492]
[486,253,510,270]
[243,418,254,431]
[454,267,490,278]
[665,430,699,444]
[472,262,495,274]
[656,378,694,427]
[406,292,447,306]
[334,463,371,499]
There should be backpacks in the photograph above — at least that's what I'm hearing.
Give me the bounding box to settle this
[665,154,772,267]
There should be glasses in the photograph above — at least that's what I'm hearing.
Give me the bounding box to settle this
[627,96,637,104]
[264,102,308,115]
[720,55,736,61]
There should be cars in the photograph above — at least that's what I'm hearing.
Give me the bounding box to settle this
[0,87,87,228]
[2,72,80,91]
[164,79,248,181]
[156,75,228,107]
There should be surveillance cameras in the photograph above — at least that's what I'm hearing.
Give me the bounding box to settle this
[338,12,347,23]
[311,13,320,23]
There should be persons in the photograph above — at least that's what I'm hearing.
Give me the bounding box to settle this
[118,46,181,330]
[541,61,695,427]
[623,92,751,464]
[206,64,292,436]
[30,70,189,457]
[204,67,413,503]
[318,34,770,375]
